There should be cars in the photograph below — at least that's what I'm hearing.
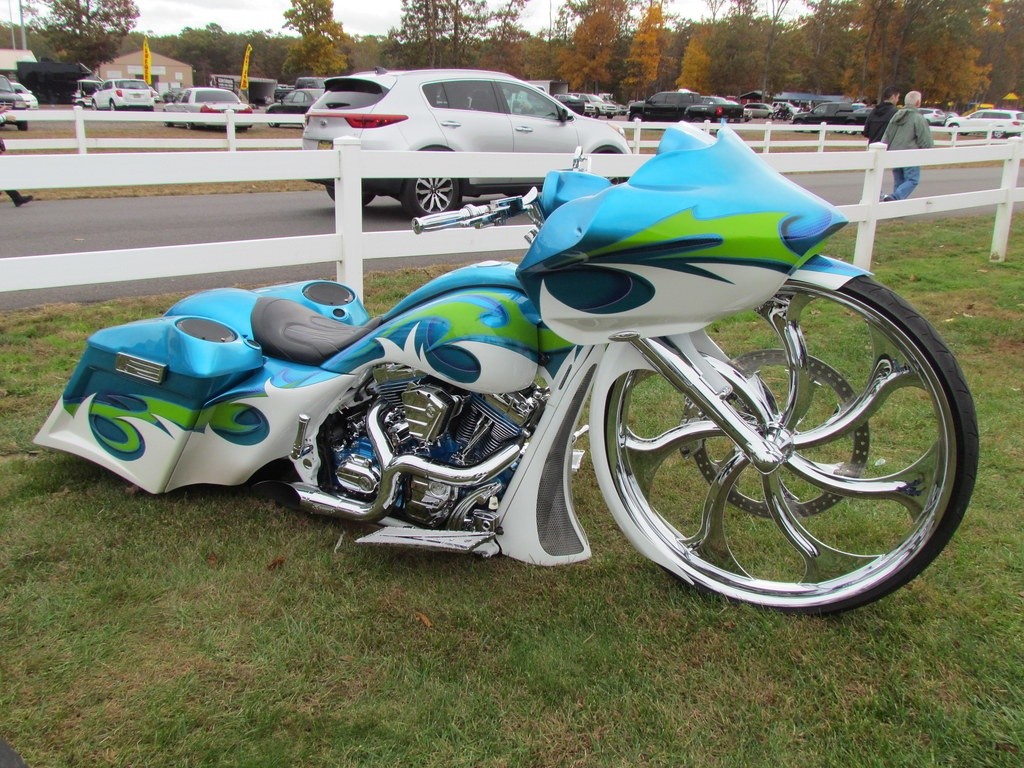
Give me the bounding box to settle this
[148,86,160,103]
[163,88,253,130]
[553,93,596,118]
[11,83,38,110]
[850,103,865,111]
[772,102,801,117]
[854,108,872,114]
[700,96,752,123]
[919,109,944,125]
[741,103,775,119]
[73,79,102,109]
[570,93,618,118]
[91,79,155,111]
[265,89,326,127]
[605,100,628,115]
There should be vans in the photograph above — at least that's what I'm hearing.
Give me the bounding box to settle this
[274,87,294,102]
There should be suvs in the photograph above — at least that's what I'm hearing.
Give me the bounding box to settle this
[946,110,1024,139]
[299,69,635,215]
[0,75,28,131]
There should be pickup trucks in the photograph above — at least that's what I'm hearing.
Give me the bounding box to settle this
[792,102,869,135]
[628,92,745,123]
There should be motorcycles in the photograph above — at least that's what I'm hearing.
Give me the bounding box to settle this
[34,120,977,621]
[772,110,792,120]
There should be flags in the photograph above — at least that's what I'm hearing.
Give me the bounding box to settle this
[241,44,252,90]
[143,36,151,85]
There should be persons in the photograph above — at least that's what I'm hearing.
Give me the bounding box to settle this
[879,92,934,202]
[0,106,33,206]
[804,103,815,112]
[863,86,900,148]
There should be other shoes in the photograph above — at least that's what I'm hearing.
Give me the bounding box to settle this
[14,195,33,207]
[880,193,887,200]
[885,195,895,201]
